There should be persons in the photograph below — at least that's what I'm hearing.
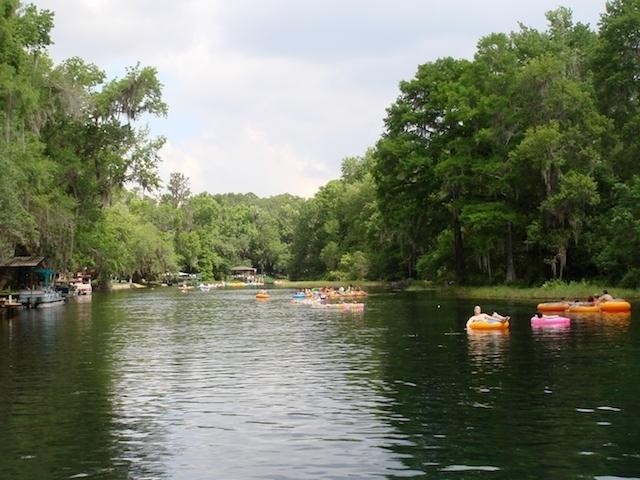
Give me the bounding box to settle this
[465,304,512,330]
[599,289,614,302]
[535,312,560,319]
[295,283,369,295]
[594,294,600,301]
[588,296,595,302]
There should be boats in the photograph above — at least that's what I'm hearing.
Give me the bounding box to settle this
[0,282,92,309]
[257,293,271,299]
[288,291,369,302]
[536,299,631,313]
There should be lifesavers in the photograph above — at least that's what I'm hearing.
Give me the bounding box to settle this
[469,317,510,330]
[256,292,270,297]
[537,299,631,313]
[532,313,569,325]
[294,287,368,297]
